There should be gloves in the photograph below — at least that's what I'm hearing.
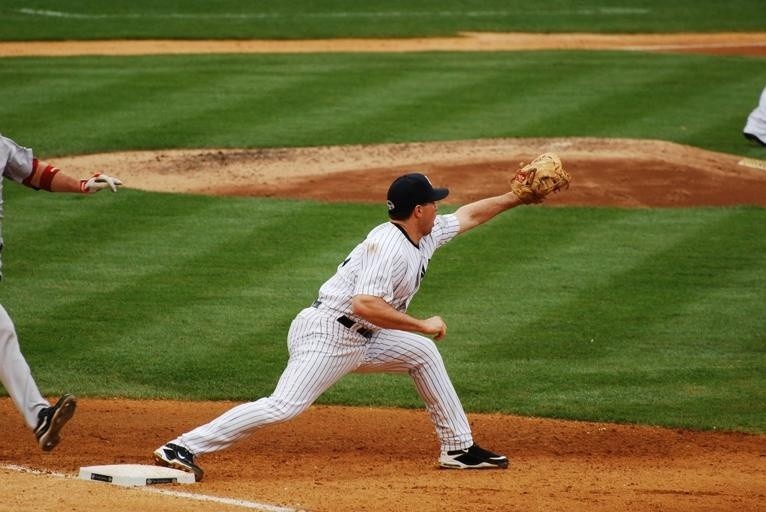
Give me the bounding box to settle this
[81,172,123,197]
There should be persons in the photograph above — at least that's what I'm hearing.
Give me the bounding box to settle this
[0,136,122,451]
[153,152,569,480]
[742,88,766,146]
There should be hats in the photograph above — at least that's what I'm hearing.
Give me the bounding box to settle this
[386,172,449,213]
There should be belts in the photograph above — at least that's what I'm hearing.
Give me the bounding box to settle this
[308,301,374,342]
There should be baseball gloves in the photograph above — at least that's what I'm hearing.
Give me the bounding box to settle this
[511,153,570,204]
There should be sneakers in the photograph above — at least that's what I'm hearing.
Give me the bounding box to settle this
[437,443,509,475]
[30,392,77,453]
[153,441,204,486]
[742,126,766,149]
